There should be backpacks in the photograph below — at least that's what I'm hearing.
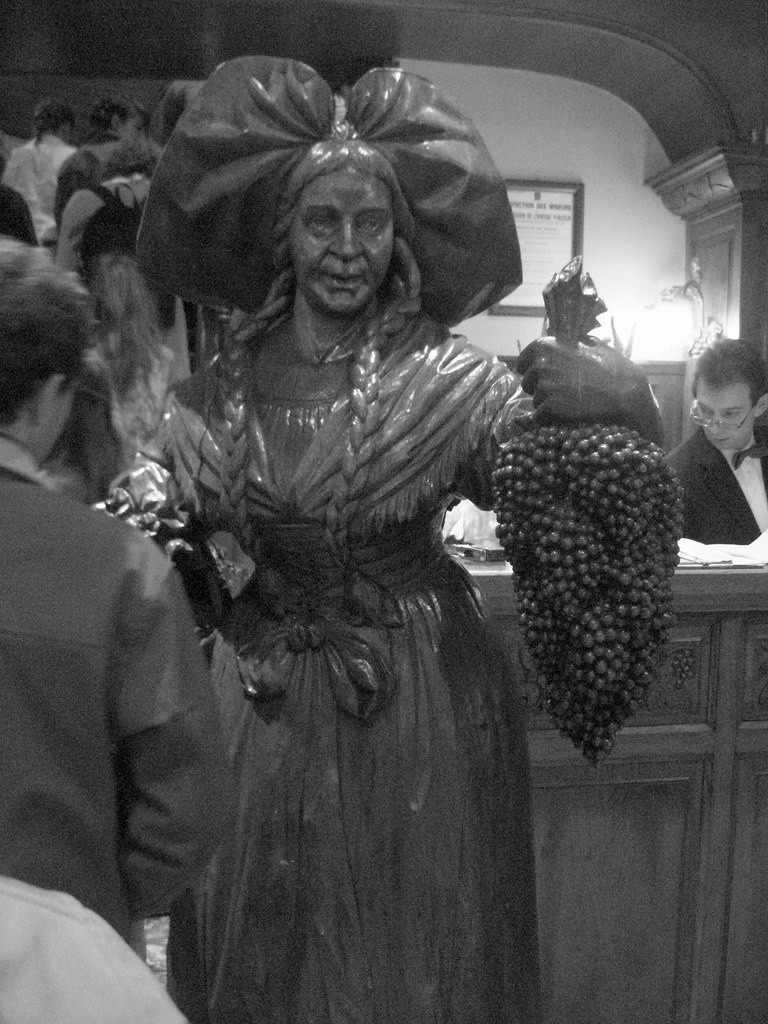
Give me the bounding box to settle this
[80,181,176,328]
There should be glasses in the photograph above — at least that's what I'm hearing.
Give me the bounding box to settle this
[689,403,756,431]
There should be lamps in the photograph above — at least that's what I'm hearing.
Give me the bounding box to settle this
[645,281,722,357]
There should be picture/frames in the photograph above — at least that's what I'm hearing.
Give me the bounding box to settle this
[488,179,585,318]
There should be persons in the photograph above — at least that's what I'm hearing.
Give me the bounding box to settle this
[0,92,196,510]
[665,341,768,544]
[137,55,664,1024]
[0,238,228,962]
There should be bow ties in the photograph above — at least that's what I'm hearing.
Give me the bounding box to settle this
[732,443,768,470]
